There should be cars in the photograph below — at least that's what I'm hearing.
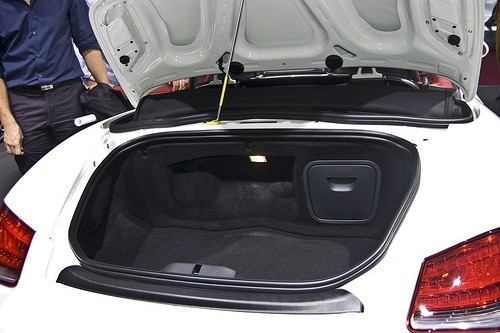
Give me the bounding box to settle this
[1,0,499,333]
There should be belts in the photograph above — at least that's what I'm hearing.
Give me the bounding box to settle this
[12,77,81,92]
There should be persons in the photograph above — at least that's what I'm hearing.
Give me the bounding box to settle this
[0,0,112,179]
[70,0,216,101]
